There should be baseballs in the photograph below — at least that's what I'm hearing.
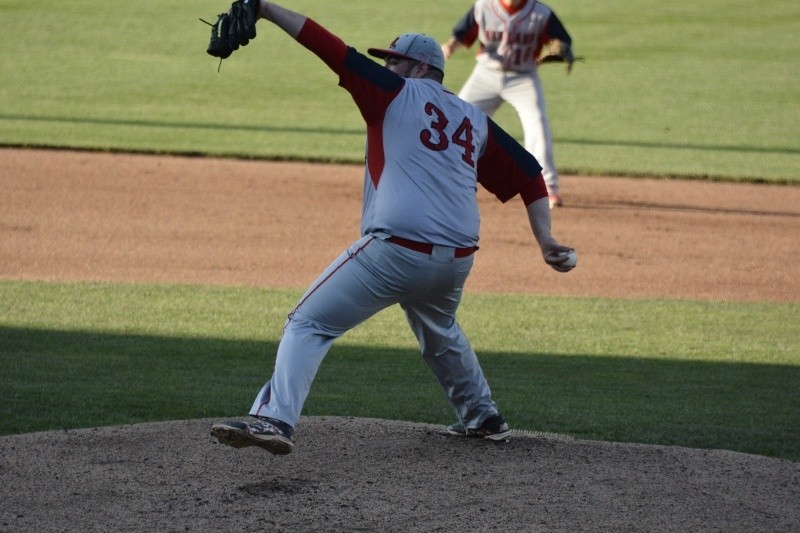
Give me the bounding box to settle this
[557,245,577,266]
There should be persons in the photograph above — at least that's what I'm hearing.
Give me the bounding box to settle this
[198,0,577,456]
[442,1,573,208]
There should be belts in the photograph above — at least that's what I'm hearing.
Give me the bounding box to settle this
[386,237,478,258]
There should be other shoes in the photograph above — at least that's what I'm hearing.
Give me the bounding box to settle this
[548,194,562,209]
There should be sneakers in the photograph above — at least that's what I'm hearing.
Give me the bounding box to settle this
[210,415,295,456]
[448,414,510,442]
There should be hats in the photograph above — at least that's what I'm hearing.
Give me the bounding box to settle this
[368,33,443,74]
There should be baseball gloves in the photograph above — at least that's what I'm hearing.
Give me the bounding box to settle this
[535,40,574,75]
[207,0,261,59]
